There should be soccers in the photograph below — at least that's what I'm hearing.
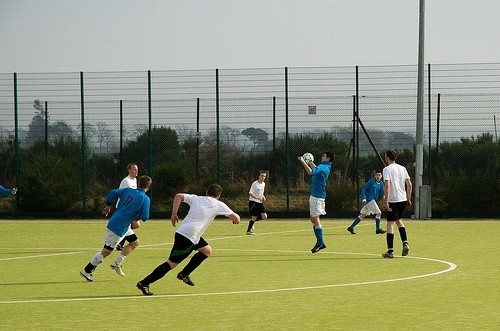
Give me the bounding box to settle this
[302,152,314,165]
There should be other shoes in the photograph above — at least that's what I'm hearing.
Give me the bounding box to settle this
[312,243,326,253]
[311,243,318,251]
[116,246,124,251]
[347,227,355,234]
[249,221,254,230]
[246,231,255,236]
[376,229,387,234]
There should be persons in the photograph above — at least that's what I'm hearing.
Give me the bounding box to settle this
[382,149,412,258]
[346,170,387,235]
[0,184,18,198]
[298,152,333,253]
[80,175,152,282]
[116,163,139,251]
[136,184,240,296]
[247,170,268,234]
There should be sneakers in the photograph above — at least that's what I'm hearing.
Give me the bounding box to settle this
[136,281,153,295]
[177,272,195,286]
[110,262,125,276]
[402,244,410,256]
[80,269,95,282]
[382,249,394,258]
